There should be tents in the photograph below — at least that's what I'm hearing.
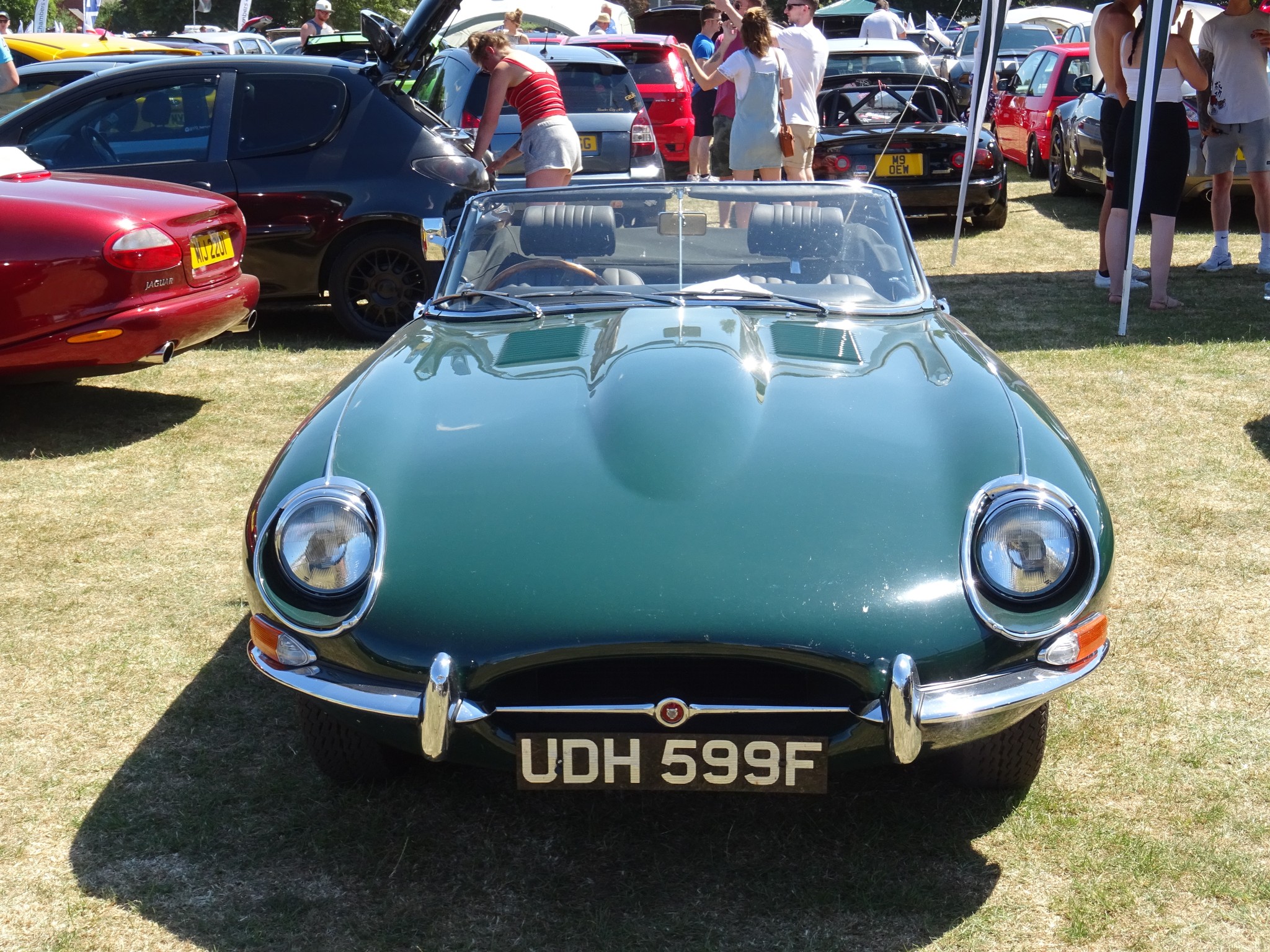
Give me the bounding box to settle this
[1004,5,1093,29]
[956,20,968,28]
[917,15,964,31]
[813,0,904,40]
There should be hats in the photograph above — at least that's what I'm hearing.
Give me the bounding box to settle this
[315,0,335,12]
[0,11,10,19]
[596,13,612,23]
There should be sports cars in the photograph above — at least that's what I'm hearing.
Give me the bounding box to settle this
[240,178,1117,797]
[0,145,263,391]
[0,0,1249,341]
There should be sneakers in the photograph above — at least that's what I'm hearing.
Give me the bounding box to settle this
[687,174,702,182]
[1126,264,1150,279]
[1196,245,1234,271]
[1094,270,1149,290]
[699,175,719,182]
[1256,251,1270,274]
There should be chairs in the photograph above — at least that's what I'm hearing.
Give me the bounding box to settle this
[141,91,171,127]
[494,205,644,288]
[728,204,875,289]
[891,91,959,123]
[816,91,863,126]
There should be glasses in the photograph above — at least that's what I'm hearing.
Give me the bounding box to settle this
[786,3,810,11]
[480,57,491,74]
[0,20,8,23]
[731,0,752,11]
[704,18,723,22]
[318,10,332,15]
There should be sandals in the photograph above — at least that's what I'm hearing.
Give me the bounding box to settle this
[1108,293,1126,304]
[1149,296,1184,310]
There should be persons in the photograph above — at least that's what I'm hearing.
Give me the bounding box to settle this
[467,31,583,189]
[0,11,20,94]
[300,0,335,46]
[170,26,271,42]
[588,0,1270,309]
[503,8,530,45]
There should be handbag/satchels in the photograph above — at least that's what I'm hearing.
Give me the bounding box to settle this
[779,125,794,157]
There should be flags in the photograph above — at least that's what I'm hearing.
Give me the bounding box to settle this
[16,0,102,34]
[237,0,252,32]
[925,10,941,32]
[907,13,916,31]
[196,0,212,13]
